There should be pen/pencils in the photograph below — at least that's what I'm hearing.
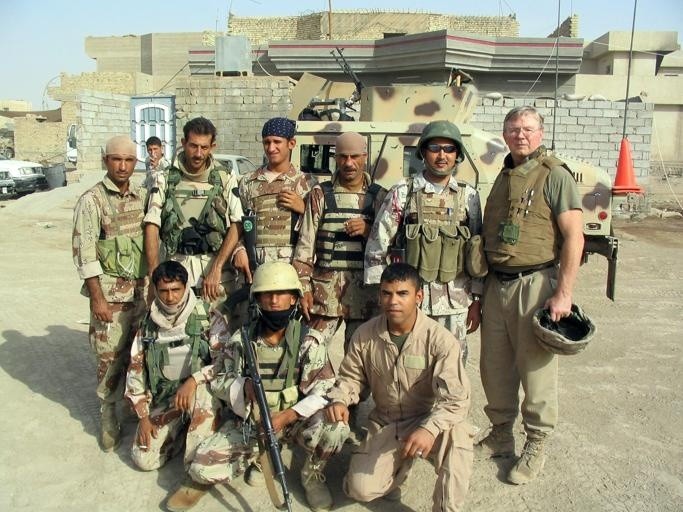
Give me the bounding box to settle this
[524,189,535,217]
[448,208,451,226]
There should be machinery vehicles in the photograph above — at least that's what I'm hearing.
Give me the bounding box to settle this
[287,45,622,307]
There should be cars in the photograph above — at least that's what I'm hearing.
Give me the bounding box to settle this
[211,153,260,178]
[0,167,18,199]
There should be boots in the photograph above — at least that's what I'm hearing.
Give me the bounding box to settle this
[384,450,417,501]
[99,398,123,453]
[165,477,212,512]
[120,399,139,424]
[472,423,514,464]
[245,441,282,487]
[299,453,335,512]
[506,436,544,486]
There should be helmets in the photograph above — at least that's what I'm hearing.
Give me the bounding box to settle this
[249,260,304,303]
[414,120,464,163]
[531,303,596,356]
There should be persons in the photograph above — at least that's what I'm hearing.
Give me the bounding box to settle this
[472,105,586,486]
[70,133,148,454]
[164,260,351,511]
[140,115,245,334]
[325,262,474,512]
[362,119,482,437]
[140,136,171,187]
[230,116,319,287]
[124,260,231,475]
[290,130,390,431]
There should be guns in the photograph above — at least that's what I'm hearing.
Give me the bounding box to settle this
[241,330,292,512]
[223,215,260,321]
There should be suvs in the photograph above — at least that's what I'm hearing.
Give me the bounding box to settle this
[0,152,45,199]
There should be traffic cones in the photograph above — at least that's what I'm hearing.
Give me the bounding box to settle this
[611,138,642,194]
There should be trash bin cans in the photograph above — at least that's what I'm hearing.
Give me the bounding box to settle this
[41,163,67,190]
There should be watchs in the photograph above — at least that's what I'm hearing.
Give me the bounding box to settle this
[473,296,481,301]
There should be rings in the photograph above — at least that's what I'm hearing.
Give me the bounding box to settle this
[416,448,423,455]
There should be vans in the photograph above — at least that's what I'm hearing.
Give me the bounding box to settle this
[66,123,77,164]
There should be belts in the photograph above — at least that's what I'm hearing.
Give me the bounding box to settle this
[490,259,557,282]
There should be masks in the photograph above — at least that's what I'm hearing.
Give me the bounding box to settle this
[256,298,300,332]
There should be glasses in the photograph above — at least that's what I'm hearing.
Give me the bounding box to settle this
[425,143,457,153]
[504,127,541,135]
